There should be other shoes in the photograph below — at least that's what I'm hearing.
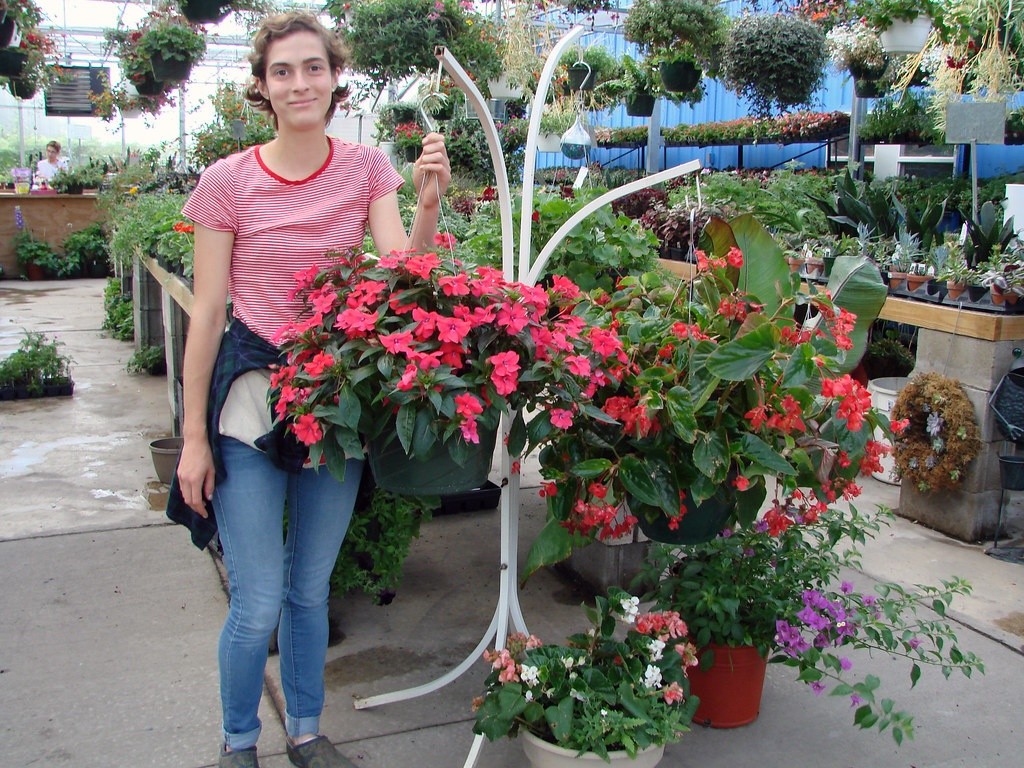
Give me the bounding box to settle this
[285,735,357,768]
[220,742,259,768]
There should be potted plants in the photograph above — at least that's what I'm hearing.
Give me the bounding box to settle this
[393,100,415,125]
[63,173,83,194]
[84,172,97,188]
[559,45,604,90]
[653,46,704,91]
[784,221,1024,309]
[83,234,110,278]
[17,232,48,281]
[621,76,657,117]
[429,98,455,121]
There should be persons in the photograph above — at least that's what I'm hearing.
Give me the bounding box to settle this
[36,141,66,180]
[175,13,450,768]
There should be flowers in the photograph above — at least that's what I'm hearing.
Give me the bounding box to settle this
[265,169,977,766]
[0,0,1023,165]
[0,331,77,387]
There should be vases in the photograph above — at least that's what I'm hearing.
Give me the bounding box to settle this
[366,410,500,493]
[854,76,886,98]
[151,49,191,81]
[0,49,24,77]
[849,53,887,77]
[149,436,183,484]
[521,726,667,768]
[630,461,738,545]
[0,382,75,399]
[879,14,930,52]
[181,0,234,24]
[135,71,164,96]
[119,93,142,118]
[0,9,15,47]
[402,139,424,162]
[559,517,654,595]
[10,77,35,99]
[683,635,769,728]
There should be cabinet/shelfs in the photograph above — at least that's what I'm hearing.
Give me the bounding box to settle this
[587,131,850,180]
